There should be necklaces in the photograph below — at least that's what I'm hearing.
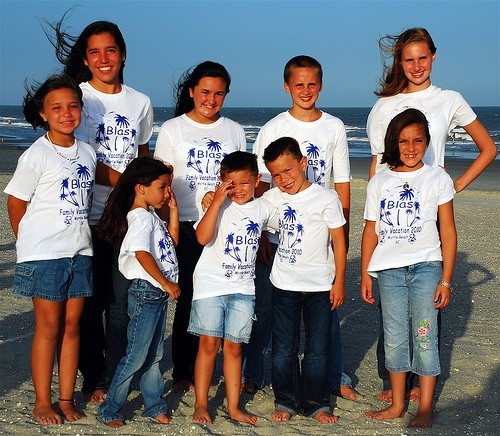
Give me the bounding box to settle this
[47,131,78,161]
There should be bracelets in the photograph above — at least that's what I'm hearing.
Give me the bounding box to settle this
[438,280,452,289]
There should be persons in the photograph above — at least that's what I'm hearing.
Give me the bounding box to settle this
[201,137,347,424]
[241,55,363,401]
[3,74,98,426]
[46,8,154,403]
[360,108,458,429]
[152,61,247,393]
[95,154,181,428]
[187,150,278,424]
[366,28,497,402]
